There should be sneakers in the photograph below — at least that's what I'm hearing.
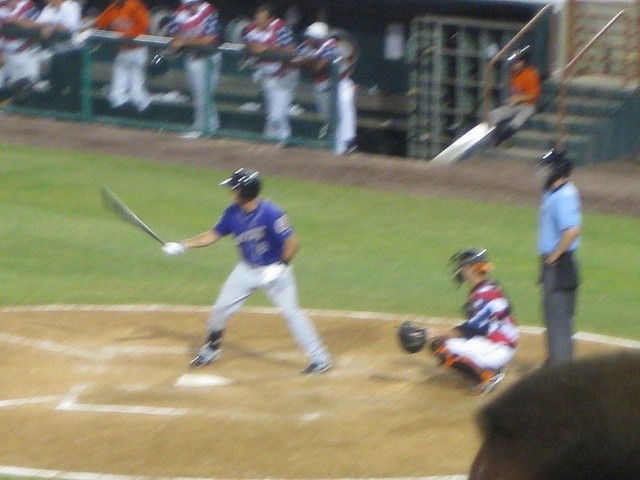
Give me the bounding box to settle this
[190,346,223,367]
[469,371,506,396]
[303,360,333,376]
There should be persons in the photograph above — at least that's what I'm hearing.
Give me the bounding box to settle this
[160,168,333,376]
[240,3,301,143]
[536,146,583,364]
[487,49,541,147]
[468,349,640,479]
[0,0,50,103]
[5,0,82,114]
[157,0,224,140]
[291,22,359,154]
[75,0,153,119]
[397,248,520,398]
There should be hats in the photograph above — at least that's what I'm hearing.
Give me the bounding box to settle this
[503,46,529,65]
[303,22,330,41]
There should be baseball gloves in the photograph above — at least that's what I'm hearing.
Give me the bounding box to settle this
[400,320,427,352]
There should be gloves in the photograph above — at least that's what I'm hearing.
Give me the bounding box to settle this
[262,262,286,284]
[163,241,188,259]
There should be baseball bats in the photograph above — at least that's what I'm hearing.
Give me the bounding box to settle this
[103,187,166,246]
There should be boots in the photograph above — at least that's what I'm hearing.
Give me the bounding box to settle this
[491,127,514,149]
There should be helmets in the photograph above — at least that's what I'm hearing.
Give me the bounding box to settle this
[543,144,576,190]
[449,248,487,284]
[143,54,170,81]
[220,168,262,201]
